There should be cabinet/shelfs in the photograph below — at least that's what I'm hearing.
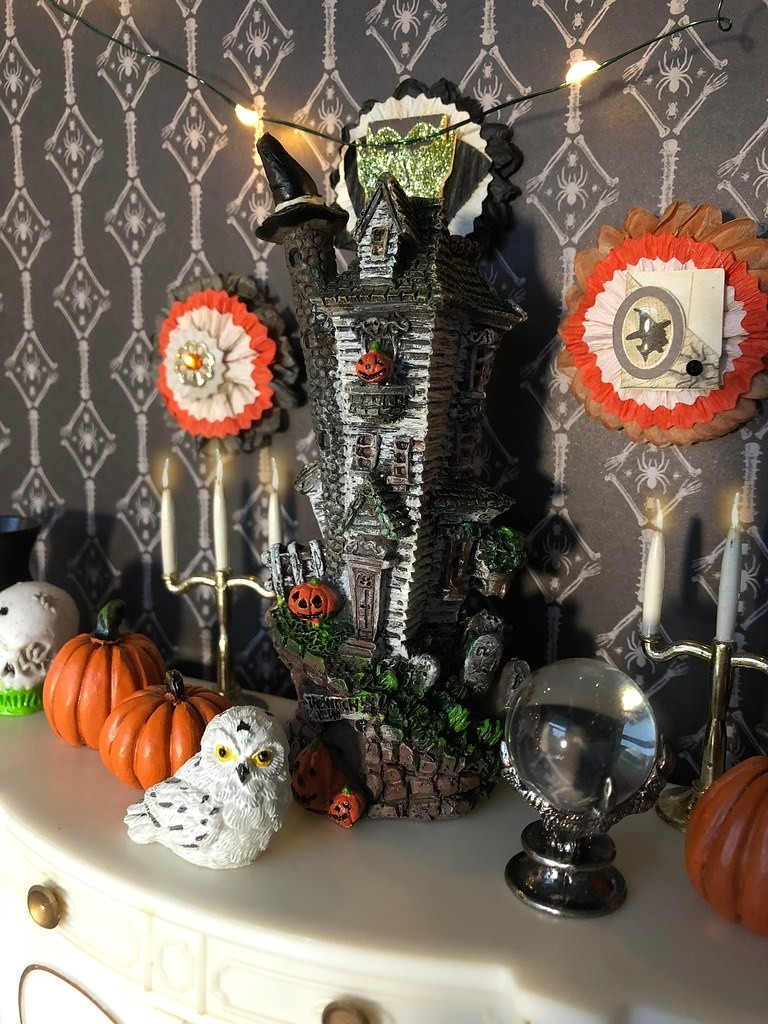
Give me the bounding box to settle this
[0,675,768,1024]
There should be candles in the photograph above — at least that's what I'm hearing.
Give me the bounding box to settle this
[160,469,175,577]
[269,469,282,545]
[213,459,228,570]
[642,510,666,635]
[715,504,741,641]
